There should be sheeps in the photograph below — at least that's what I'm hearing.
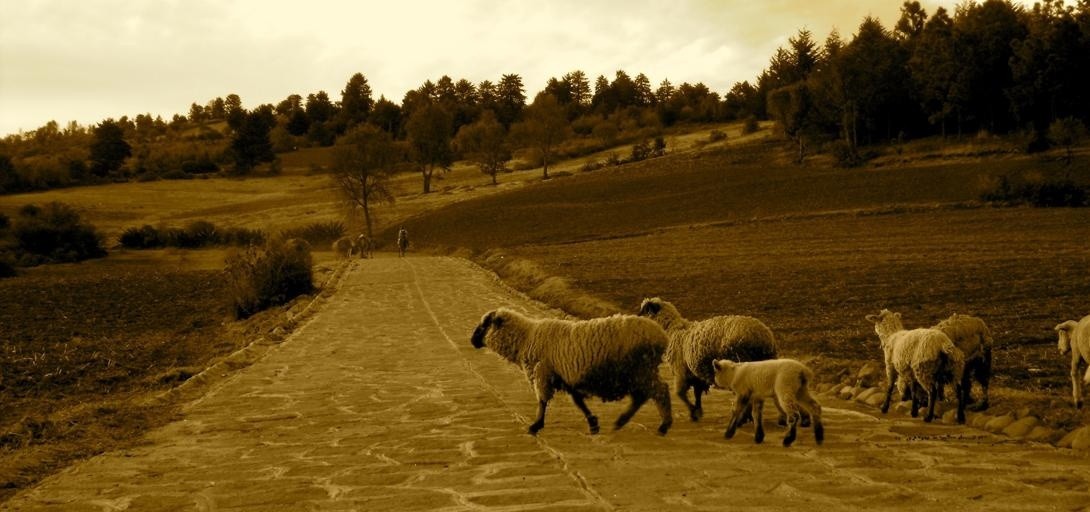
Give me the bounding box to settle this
[712,358,824,447]
[1055,314,1090,409]
[471,307,672,436]
[638,298,778,427]
[897,312,994,412]
[865,309,965,424]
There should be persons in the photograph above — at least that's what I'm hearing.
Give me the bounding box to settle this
[397,225,409,257]
